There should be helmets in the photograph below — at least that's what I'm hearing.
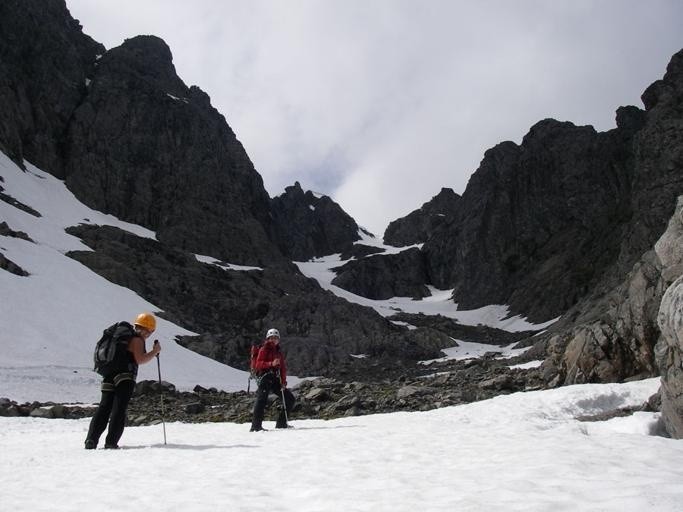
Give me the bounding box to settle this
[266,328,280,339]
[134,313,156,333]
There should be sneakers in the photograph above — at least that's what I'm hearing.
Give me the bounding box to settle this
[275,423,293,428]
[250,428,269,432]
[84,440,121,449]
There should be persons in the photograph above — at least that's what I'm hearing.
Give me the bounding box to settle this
[247,328,295,433]
[83,311,161,449]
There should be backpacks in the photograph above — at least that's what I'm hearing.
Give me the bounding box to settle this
[249,342,261,379]
[93,318,133,366]
